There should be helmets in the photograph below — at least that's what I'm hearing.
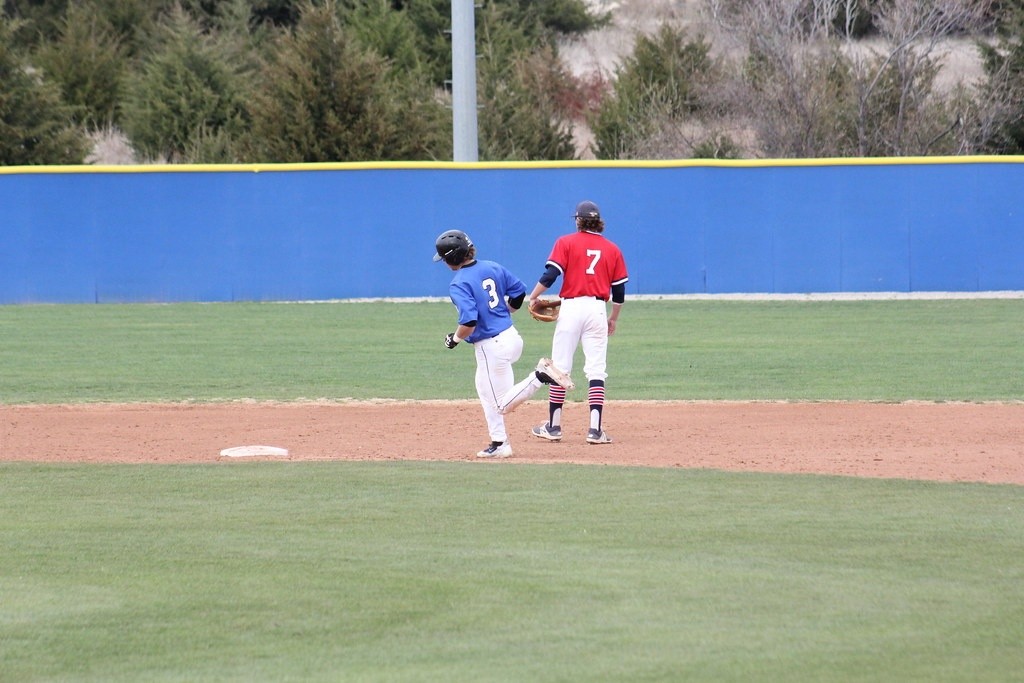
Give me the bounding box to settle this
[432,230,473,262]
[569,200,600,218]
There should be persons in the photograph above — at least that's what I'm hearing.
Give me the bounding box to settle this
[433,230,574,459]
[529,201,628,445]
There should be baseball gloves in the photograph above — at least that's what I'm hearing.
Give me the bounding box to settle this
[527,299,562,322]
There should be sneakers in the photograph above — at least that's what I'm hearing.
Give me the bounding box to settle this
[535,357,576,391]
[477,440,513,458]
[532,422,562,442]
[586,428,613,444]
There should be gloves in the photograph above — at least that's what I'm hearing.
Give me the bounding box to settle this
[444,332,462,349]
